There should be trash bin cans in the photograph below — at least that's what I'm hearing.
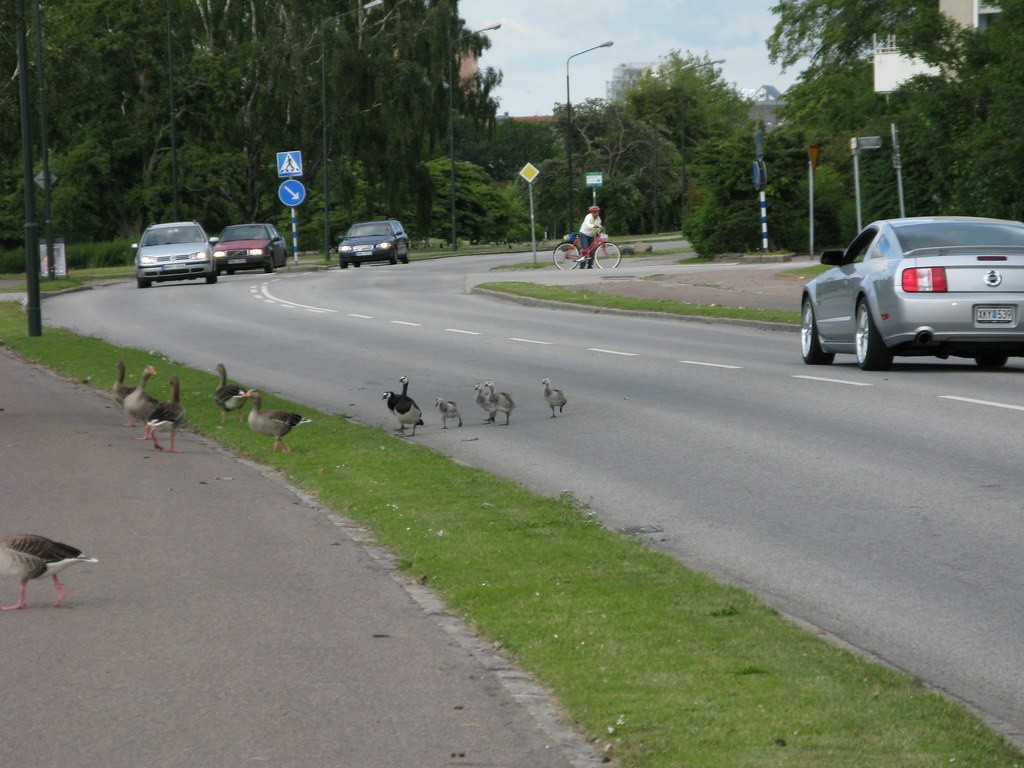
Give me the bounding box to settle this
[38,235,68,280]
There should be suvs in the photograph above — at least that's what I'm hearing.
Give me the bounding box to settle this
[337,218,412,271]
[130,219,220,289]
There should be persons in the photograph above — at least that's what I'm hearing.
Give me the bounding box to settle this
[578,206,602,270]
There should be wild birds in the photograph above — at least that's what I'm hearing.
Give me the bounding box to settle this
[474,379,515,426]
[435,398,463,429]
[541,378,567,419]
[382,376,425,436]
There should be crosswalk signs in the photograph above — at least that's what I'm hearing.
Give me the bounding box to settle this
[277,150,304,178]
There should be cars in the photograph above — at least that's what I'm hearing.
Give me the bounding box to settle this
[798,216,1024,372]
[214,223,289,276]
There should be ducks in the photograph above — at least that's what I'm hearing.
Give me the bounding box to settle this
[213,362,313,453]
[0,534,99,610]
[112,359,185,453]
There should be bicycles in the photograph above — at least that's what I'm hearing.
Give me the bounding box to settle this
[551,226,622,270]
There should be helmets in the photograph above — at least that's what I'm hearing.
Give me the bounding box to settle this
[588,205,600,212]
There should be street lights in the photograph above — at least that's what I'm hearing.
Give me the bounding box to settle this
[678,59,727,217]
[565,41,615,233]
[317,0,387,260]
[447,23,501,255]
[652,64,696,233]
